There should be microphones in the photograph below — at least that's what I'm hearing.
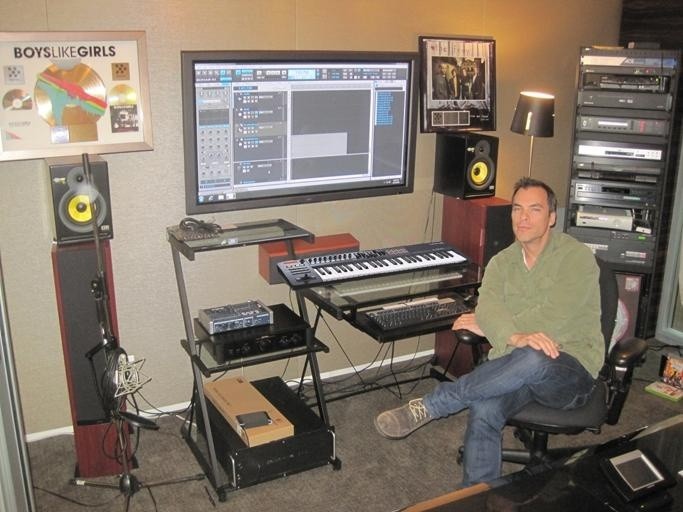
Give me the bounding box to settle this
[81,150,98,196]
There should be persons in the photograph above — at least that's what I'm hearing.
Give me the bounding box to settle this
[448,69,460,99]
[373,176,607,494]
[470,62,482,100]
[432,62,451,99]
[461,67,470,100]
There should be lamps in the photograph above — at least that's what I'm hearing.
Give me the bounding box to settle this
[510,90,554,179]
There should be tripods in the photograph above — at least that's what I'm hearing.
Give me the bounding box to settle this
[65,202,207,512]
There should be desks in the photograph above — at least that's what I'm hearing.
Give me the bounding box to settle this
[166,216,485,501]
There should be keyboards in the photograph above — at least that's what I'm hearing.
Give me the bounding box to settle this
[364,299,472,333]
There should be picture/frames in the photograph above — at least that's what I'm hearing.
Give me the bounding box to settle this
[418,34,496,133]
[0,29,153,162]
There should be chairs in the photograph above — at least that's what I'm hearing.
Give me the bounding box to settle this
[456,256,647,489]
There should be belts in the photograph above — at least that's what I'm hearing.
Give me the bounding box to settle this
[374,398,433,440]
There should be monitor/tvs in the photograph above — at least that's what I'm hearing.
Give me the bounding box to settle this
[179,49,421,214]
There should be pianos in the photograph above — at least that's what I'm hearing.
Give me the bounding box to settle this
[274,241,471,290]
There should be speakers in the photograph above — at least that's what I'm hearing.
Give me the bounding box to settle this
[43,152,115,246]
[436,195,514,378]
[49,240,137,475]
[434,128,500,199]
